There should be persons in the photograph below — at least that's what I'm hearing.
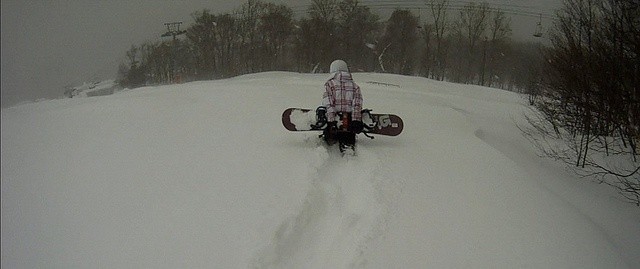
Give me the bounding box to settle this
[322,60,364,156]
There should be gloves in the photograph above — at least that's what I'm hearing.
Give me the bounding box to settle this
[327,121,338,136]
[347,121,362,134]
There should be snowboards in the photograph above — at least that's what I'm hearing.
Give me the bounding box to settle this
[282,108,404,136]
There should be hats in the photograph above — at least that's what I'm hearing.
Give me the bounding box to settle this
[330,60,348,74]
